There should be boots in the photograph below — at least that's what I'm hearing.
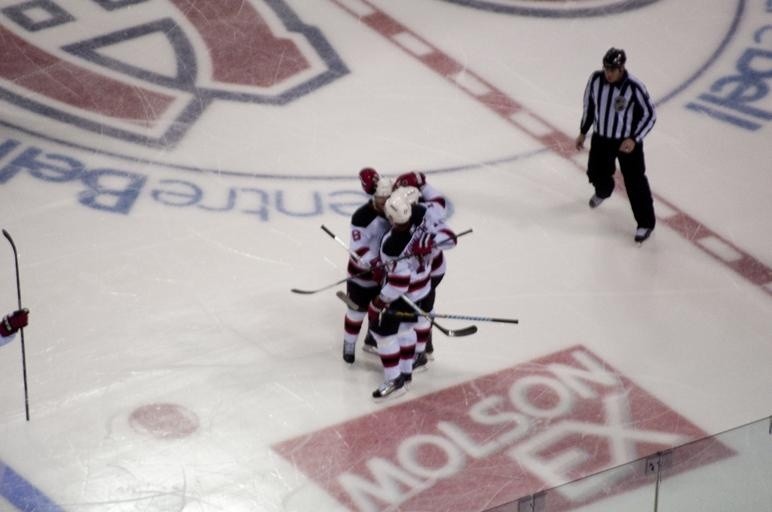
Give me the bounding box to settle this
[590,193,604,207]
[343,333,435,399]
[635,227,653,242]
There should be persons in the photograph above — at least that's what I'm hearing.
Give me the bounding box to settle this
[341,167,457,400]
[0,307,29,348]
[573,47,657,243]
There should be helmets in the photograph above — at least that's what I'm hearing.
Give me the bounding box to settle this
[374,178,424,227]
[604,47,626,69]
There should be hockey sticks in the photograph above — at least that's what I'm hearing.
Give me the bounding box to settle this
[293,229,472,295]
[336,289,520,325]
[320,224,478,336]
[1,226,29,421]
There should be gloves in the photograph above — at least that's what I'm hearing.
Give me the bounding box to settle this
[412,233,436,257]
[0,308,29,337]
[359,168,379,194]
[391,172,427,189]
[369,260,386,281]
[368,301,386,327]
